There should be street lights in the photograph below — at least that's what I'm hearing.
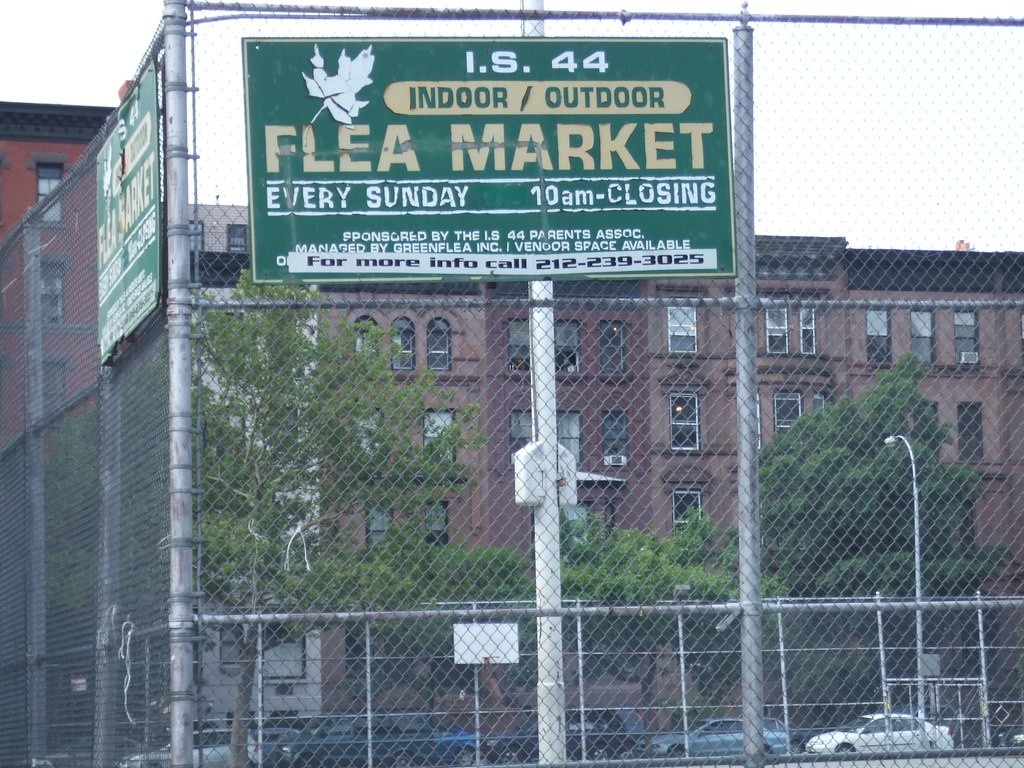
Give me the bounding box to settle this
[885,434,928,750]
[670,583,689,756]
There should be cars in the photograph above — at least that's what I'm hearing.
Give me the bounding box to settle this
[482,709,642,766]
[114,725,261,768]
[29,732,151,767]
[999,728,1024,746]
[434,723,484,767]
[250,727,303,767]
[648,717,789,754]
[804,712,955,753]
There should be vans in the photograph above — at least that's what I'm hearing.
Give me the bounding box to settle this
[262,708,442,767]
